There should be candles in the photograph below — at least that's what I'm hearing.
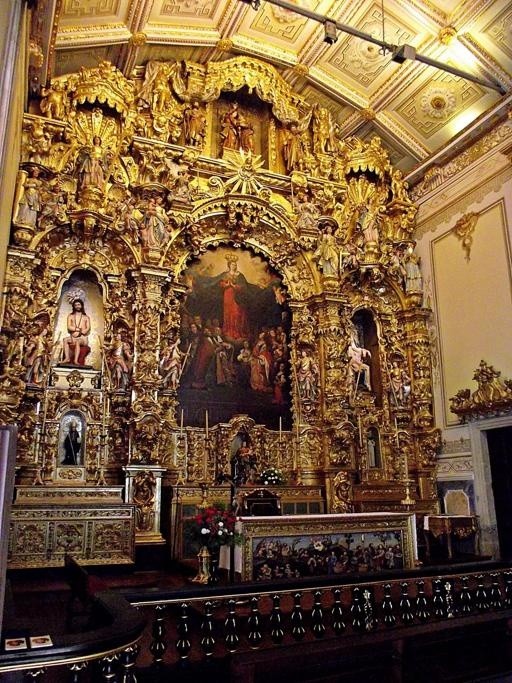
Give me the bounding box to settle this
[206,409,209,438]
[296,418,299,441]
[279,416,282,441]
[180,408,184,438]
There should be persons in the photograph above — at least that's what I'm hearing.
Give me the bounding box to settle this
[135,468,157,532]
[431,523,476,555]
[236,439,254,478]
[136,318,157,341]
[390,170,415,204]
[15,164,43,230]
[252,534,403,584]
[219,101,245,156]
[316,108,333,156]
[387,362,412,406]
[55,297,92,366]
[366,435,378,467]
[349,332,372,391]
[290,307,318,398]
[100,332,134,388]
[297,188,343,278]
[163,337,190,391]
[136,171,189,248]
[184,102,207,145]
[226,206,263,232]
[144,115,170,143]
[241,156,256,174]
[24,324,54,386]
[60,418,83,466]
[282,106,306,172]
[77,134,118,195]
[152,67,176,111]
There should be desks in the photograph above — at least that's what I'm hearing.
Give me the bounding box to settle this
[422,513,480,559]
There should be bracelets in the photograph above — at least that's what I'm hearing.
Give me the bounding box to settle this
[75,327,77,331]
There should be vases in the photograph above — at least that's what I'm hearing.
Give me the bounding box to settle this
[193,544,212,583]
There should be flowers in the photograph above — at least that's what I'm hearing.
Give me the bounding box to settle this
[181,507,246,548]
[260,466,288,485]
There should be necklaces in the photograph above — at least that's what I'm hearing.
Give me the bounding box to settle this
[74,312,83,328]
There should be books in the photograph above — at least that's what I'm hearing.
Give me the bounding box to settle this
[29,634,54,649]
[4,637,28,651]
